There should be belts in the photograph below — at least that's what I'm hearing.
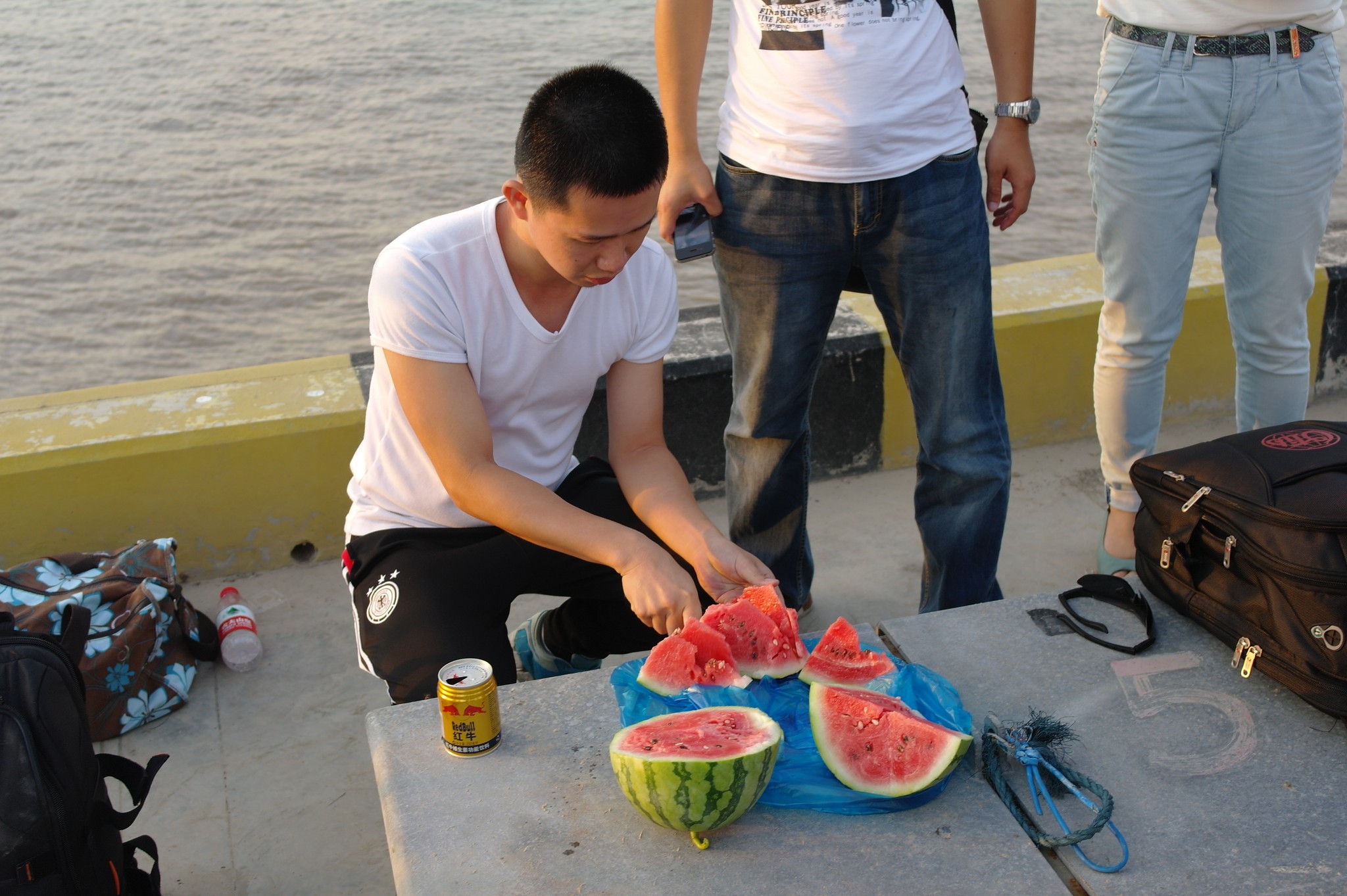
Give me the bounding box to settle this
[1106,13,1325,58]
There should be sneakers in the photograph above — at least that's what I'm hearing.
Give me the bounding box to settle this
[509,610,603,681]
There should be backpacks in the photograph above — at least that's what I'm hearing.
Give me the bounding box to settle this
[1130,419,1347,722]
[0,537,218,744]
[0,603,170,896]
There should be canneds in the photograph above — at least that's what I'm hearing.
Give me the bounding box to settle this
[438,658,502,758]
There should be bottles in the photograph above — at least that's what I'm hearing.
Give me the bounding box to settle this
[215,586,263,674]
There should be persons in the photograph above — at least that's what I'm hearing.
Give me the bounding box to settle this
[339,63,788,706]
[653,0,1039,613]
[1088,0,1347,576]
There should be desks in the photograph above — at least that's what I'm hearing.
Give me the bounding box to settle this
[364,578,1347,896]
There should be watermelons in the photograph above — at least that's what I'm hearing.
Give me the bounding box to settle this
[809,681,973,797]
[797,617,898,688]
[636,582,812,697]
[610,705,783,850]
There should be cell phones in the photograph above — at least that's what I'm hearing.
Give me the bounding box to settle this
[672,202,717,263]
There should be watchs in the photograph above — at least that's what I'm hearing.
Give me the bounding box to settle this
[994,97,1041,124]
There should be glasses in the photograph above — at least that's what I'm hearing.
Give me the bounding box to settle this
[1059,573,1156,656]
